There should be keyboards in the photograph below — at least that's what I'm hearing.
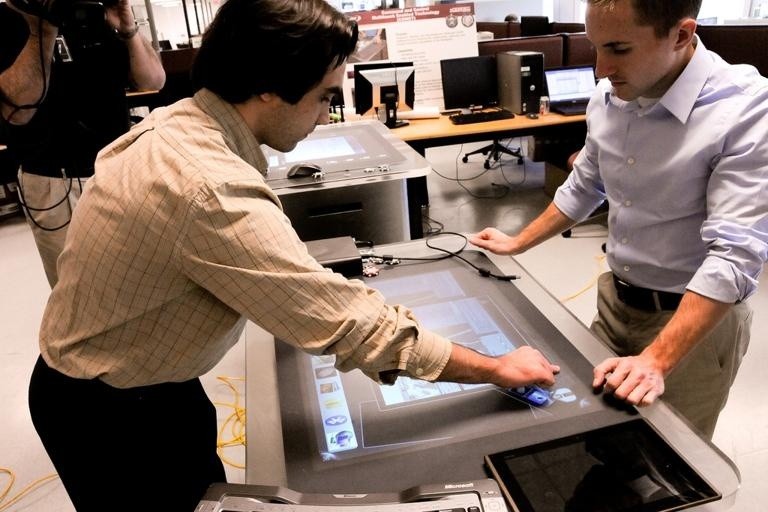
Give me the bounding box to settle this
[449,109,515,125]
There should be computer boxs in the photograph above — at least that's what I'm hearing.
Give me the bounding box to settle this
[497,50,544,115]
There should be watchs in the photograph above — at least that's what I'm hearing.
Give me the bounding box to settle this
[119,20,139,37]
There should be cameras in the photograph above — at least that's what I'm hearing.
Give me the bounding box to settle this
[1,1,118,31]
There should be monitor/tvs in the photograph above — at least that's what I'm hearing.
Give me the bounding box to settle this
[520,16,549,36]
[247,232,737,512]
[440,54,500,114]
[354,62,415,129]
[260,119,432,248]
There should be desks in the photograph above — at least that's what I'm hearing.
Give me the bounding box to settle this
[344,103,602,240]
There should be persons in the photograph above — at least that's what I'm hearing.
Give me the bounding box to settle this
[29,0,559,511]
[1,0,167,291]
[468,0,768,447]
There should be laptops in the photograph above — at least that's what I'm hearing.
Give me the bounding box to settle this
[544,64,597,116]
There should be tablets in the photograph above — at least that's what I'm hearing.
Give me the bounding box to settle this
[483,417,722,512]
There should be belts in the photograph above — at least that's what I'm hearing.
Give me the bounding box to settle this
[20,164,93,178]
[613,270,685,312]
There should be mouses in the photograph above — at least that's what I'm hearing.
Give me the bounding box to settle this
[527,113,538,119]
[287,163,322,179]
